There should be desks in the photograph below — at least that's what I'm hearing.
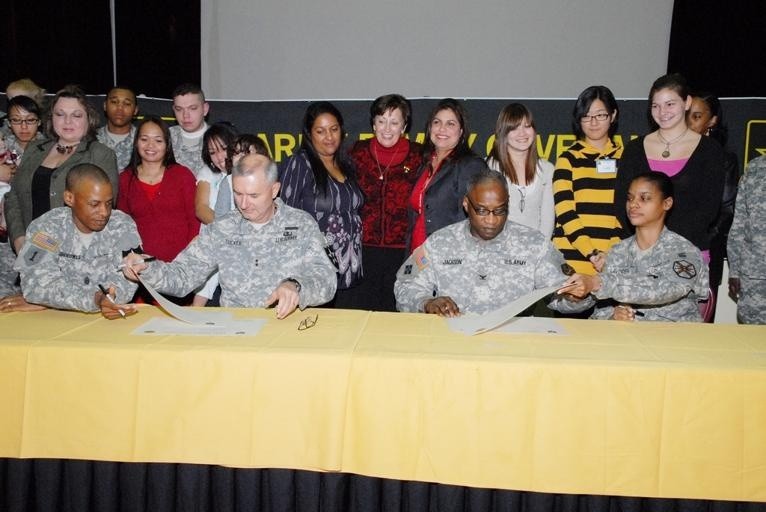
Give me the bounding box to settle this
[1,286,765,512]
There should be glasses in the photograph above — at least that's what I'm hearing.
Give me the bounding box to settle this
[468,198,507,216]
[10,118,38,124]
[581,114,609,121]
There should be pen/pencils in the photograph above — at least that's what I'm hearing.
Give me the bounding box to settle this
[98,284,125,317]
[115,257,156,268]
[618,305,644,316]
